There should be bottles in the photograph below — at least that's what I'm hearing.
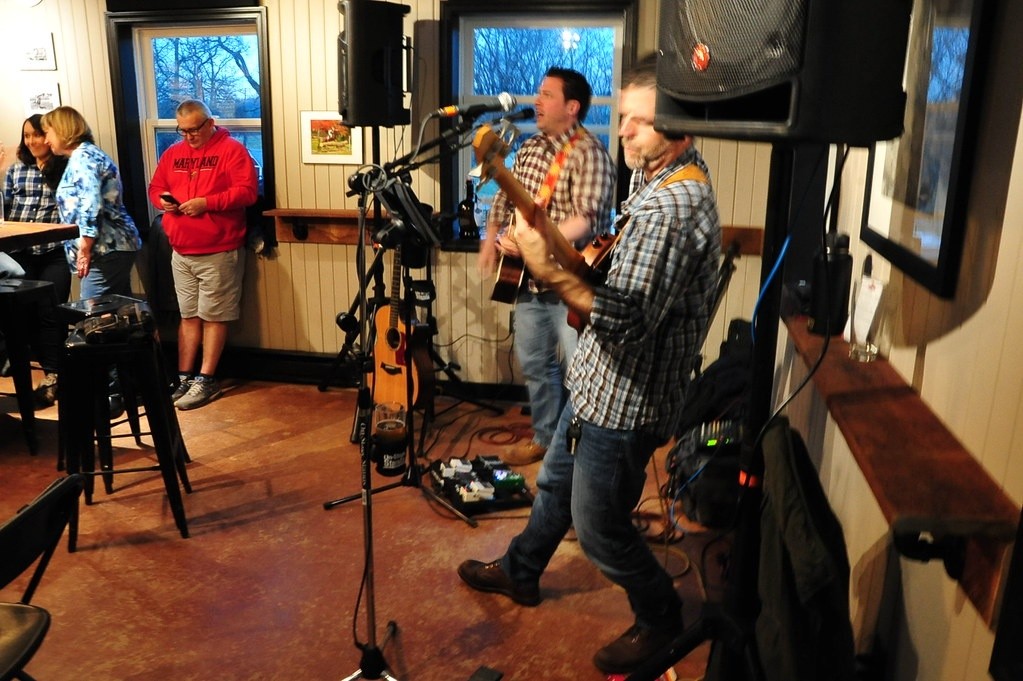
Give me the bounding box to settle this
[458,180,481,240]
[806,235,853,337]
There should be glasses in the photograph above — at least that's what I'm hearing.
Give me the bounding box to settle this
[176,118,209,136]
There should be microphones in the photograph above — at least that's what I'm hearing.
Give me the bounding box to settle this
[476,108,535,130]
[430,92,517,119]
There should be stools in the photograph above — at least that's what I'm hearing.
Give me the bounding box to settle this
[0,278,195,554]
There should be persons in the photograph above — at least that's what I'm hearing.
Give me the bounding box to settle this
[2,114,72,380]
[40,106,143,308]
[481,67,617,465]
[456,54,721,673]
[148,99,259,411]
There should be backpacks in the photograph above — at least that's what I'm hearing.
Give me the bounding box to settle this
[666,318,769,532]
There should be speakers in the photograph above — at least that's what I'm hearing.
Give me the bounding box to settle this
[653,0,915,148]
[336,0,411,128]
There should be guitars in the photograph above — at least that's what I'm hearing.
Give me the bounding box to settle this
[471,116,620,335]
[488,213,525,305]
[365,240,420,413]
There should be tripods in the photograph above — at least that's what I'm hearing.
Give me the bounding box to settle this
[317,124,478,681]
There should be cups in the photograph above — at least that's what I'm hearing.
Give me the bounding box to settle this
[374,400,408,476]
[847,279,889,361]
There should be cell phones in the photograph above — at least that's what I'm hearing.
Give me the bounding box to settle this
[160,195,182,207]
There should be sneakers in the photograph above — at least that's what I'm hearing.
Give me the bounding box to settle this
[504,440,549,464]
[458,559,540,607]
[172,376,195,402]
[592,613,684,674]
[173,378,221,410]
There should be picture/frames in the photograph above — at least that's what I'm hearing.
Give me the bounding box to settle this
[860,0,1011,301]
[301,110,364,165]
